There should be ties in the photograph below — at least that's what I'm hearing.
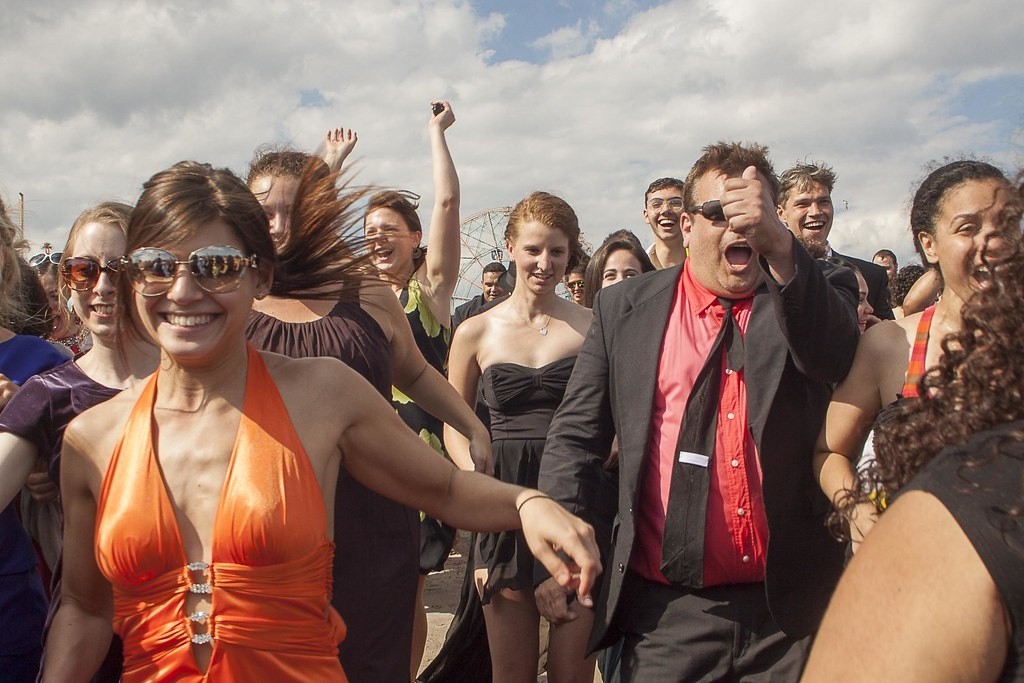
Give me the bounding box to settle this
[659,296,745,590]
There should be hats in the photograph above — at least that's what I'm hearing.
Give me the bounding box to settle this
[497,261,517,292]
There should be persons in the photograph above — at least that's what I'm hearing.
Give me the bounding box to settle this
[775,160,944,337]
[0,196,163,682]
[240,148,500,682]
[532,146,863,683]
[793,167,1023,683]
[440,187,617,683]
[319,100,462,683]
[36,158,604,683]
[810,156,1023,556]
[560,176,688,310]
[450,256,517,343]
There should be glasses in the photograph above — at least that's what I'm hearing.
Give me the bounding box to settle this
[56,257,136,296]
[119,244,258,297]
[27,252,64,267]
[686,200,726,222]
[647,198,684,212]
[566,280,585,289]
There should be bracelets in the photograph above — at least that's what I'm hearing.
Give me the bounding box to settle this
[55,488,61,503]
[515,495,558,513]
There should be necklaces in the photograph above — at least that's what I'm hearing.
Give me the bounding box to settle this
[512,292,557,335]
[43,320,90,352]
